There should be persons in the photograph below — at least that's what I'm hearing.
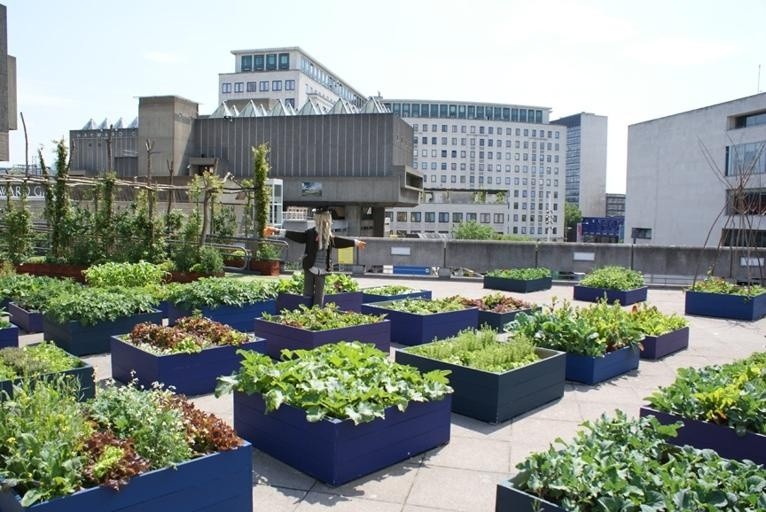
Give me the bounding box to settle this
[264,207,367,309]
